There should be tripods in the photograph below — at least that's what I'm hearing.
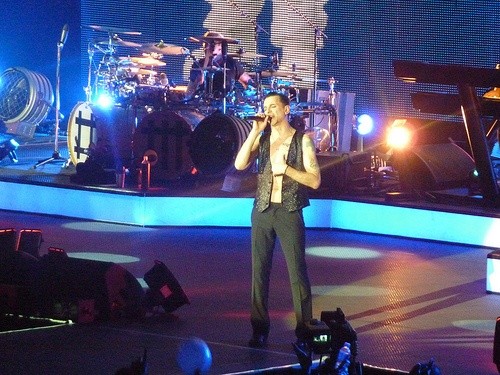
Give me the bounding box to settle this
[32,42,69,168]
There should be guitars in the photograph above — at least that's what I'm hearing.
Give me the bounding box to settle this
[349,113,360,152]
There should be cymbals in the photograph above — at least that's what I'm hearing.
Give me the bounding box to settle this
[225,52,268,58]
[118,56,166,66]
[136,43,190,55]
[288,66,309,71]
[110,67,159,75]
[96,38,143,48]
[79,22,143,35]
[247,71,297,77]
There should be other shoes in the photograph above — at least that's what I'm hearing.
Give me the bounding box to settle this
[249,334,267,348]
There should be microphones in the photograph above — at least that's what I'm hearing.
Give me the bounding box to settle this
[244,116,271,120]
[60,24,69,46]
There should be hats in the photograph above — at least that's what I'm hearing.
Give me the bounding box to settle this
[187,32,237,47]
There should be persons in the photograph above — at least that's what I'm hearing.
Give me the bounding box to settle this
[235,91,322,347]
[351,358,439,375]
[290,341,315,375]
[115,346,149,375]
[184,31,254,104]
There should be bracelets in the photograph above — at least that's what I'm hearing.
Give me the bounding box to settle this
[283,164,289,175]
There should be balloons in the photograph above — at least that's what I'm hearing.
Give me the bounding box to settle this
[175,335,213,374]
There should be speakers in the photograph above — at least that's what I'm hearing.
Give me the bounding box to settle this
[33,253,142,323]
[398,142,476,199]
[310,150,371,194]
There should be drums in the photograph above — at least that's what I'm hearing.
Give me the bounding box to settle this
[135,84,165,105]
[67,101,112,169]
[166,83,187,108]
[189,111,256,180]
[131,110,207,187]
